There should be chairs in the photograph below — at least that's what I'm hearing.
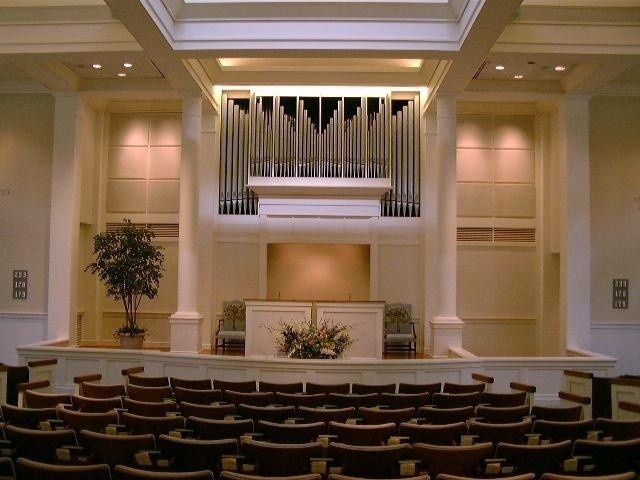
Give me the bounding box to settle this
[383,303,417,359]
[214,300,246,355]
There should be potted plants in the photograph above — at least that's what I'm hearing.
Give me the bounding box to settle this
[83,217,168,349]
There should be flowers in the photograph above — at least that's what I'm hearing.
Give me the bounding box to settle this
[257,314,357,359]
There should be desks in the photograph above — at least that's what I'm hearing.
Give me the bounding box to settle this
[243,298,313,357]
[316,299,385,359]
